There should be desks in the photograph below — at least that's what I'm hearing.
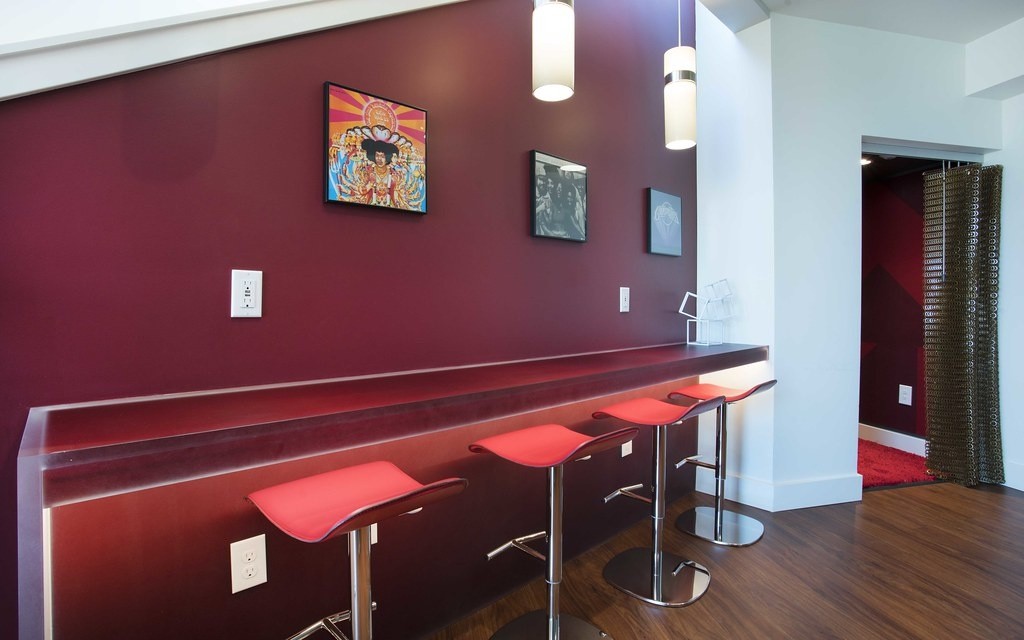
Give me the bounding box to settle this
[17,344,769,640]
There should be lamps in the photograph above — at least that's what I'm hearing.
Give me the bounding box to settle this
[663,0,697,151]
[532,0,575,102]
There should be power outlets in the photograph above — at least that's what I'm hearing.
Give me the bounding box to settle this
[230,532,267,595]
[230,269,263,318]
[898,384,912,406]
[620,287,630,312]
[622,440,632,457]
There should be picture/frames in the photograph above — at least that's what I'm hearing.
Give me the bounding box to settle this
[647,187,682,256]
[323,81,428,215]
[530,150,588,242]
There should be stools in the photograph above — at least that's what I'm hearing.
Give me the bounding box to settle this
[667,379,777,547]
[245,461,468,640]
[468,424,640,640]
[592,395,726,608]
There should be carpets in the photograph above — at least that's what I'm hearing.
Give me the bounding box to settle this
[858,438,944,491]
